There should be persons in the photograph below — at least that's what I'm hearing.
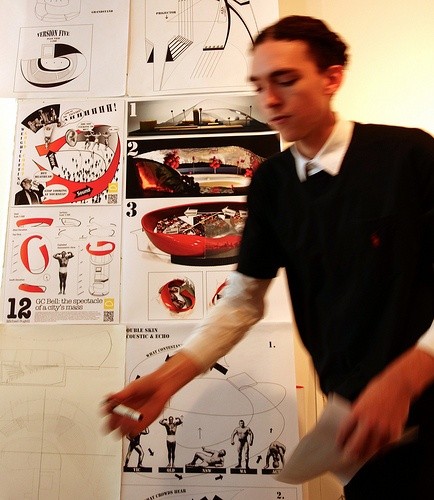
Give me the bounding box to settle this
[54,249,74,293]
[96,15,434,500]
[24,99,116,157]
[230,419,254,470]
[262,439,288,473]
[160,415,183,468]
[185,444,225,471]
[124,420,149,472]
[14,178,44,204]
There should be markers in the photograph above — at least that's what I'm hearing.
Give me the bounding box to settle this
[110,402,144,423]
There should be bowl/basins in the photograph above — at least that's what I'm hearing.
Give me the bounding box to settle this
[140,199,249,266]
